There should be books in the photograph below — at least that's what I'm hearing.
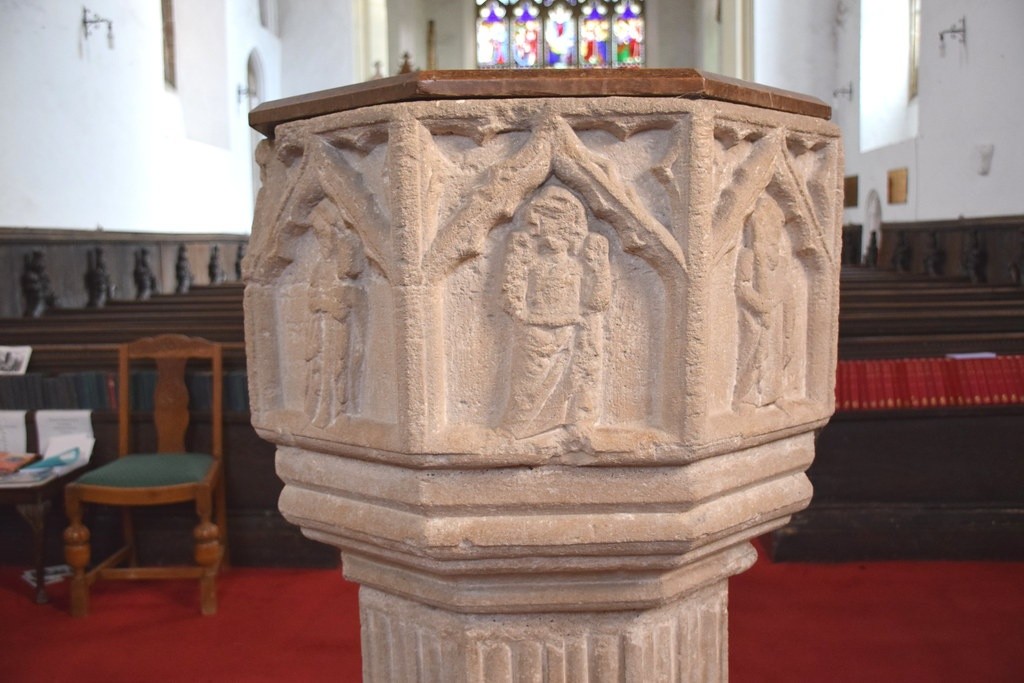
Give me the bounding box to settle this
[20,565,76,604]
[0,451,54,481]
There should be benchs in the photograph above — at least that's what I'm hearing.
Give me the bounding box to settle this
[1,272,1024,561]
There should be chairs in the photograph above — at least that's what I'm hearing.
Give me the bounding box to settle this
[65,334,227,614]
